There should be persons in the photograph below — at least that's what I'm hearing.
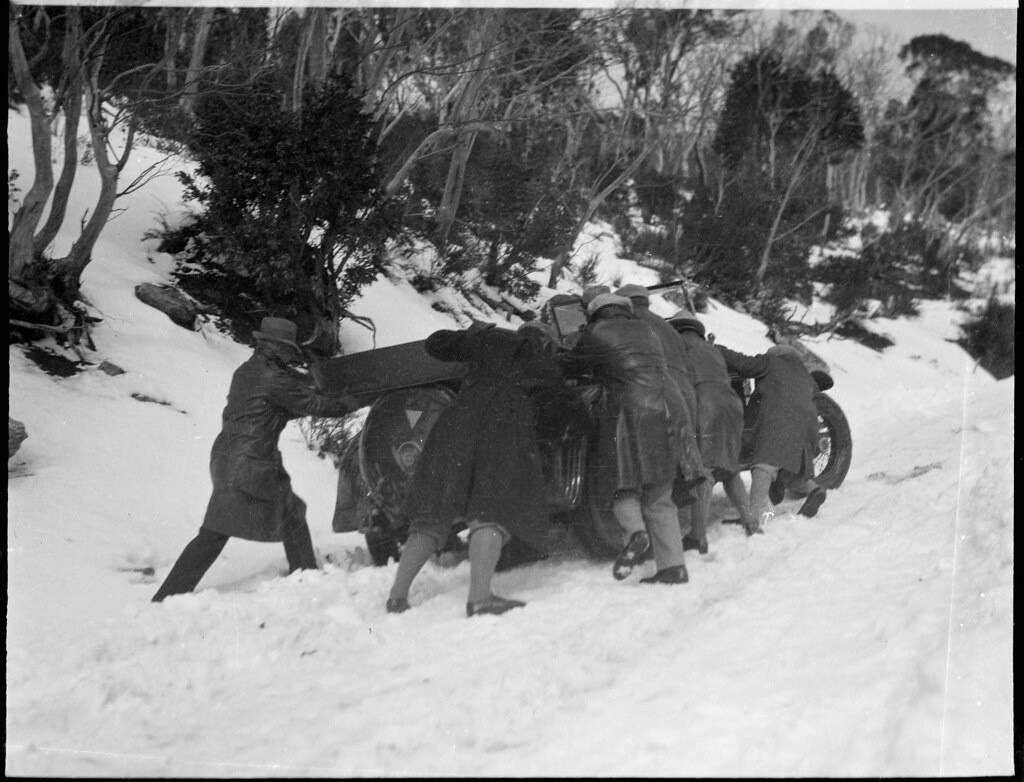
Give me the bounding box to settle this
[386,284,826,617]
[150,317,374,602]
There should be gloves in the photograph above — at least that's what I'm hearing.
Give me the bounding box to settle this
[467,320,497,332]
[340,394,360,413]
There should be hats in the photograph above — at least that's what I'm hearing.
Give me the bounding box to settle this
[767,346,803,360]
[587,293,634,316]
[257,316,305,351]
[665,311,705,335]
[616,283,650,299]
[519,321,558,342]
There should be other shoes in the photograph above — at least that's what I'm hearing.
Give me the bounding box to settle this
[797,486,827,518]
[745,519,763,535]
[467,595,526,616]
[722,516,747,525]
[638,564,691,585]
[386,598,410,612]
[612,530,649,580]
[680,532,708,555]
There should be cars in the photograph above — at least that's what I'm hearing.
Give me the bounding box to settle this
[310,279,854,563]
